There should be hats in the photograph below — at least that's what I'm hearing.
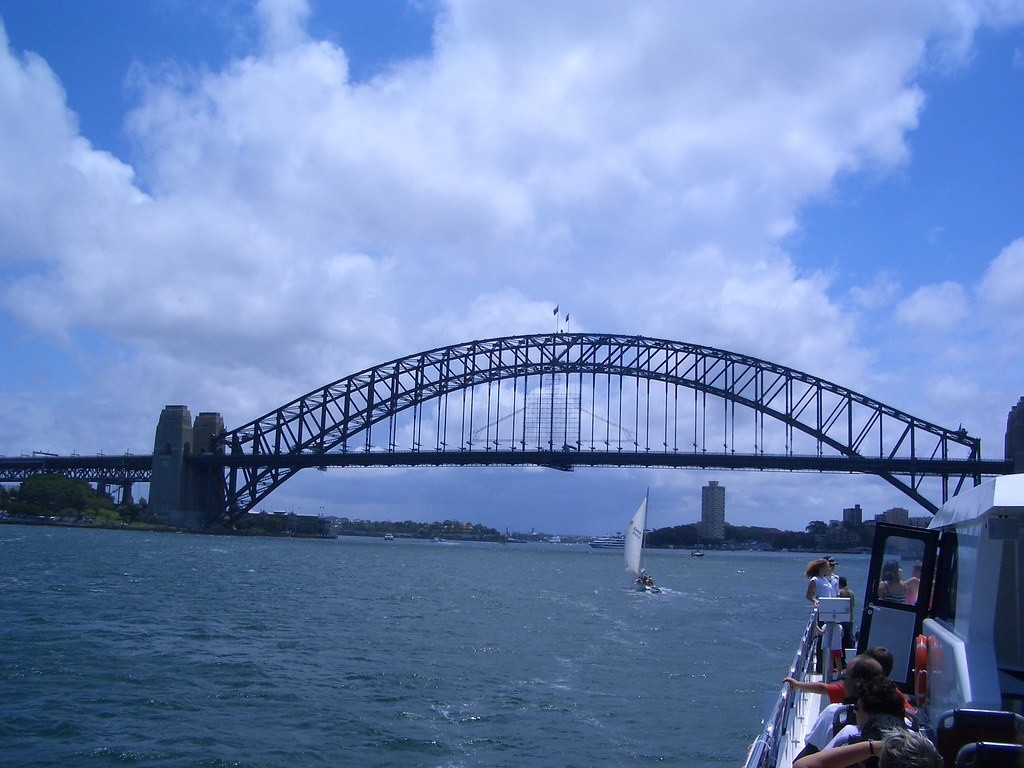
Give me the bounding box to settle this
[823,555,838,566]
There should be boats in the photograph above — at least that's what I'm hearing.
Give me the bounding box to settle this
[384,533,393,540]
[741,475,1024,768]
[691,551,705,556]
[588,531,626,549]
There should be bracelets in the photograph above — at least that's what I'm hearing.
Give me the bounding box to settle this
[868,738,874,755]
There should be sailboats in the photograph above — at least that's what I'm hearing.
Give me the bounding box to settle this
[623,487,661,593]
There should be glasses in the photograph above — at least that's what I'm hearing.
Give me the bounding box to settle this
[840,673,854,681]
[853,703,865,711]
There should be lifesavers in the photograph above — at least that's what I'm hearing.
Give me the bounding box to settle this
[914,634,928,708]
[929,633,945,702]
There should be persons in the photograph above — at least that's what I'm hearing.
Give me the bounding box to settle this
[636,574,653,586]
[805,555,855,681]
[879,560,935,608]
[782,648,945,768]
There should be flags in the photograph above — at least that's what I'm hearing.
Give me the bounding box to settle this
[553,304,559,315]
[566,313,569,322]
